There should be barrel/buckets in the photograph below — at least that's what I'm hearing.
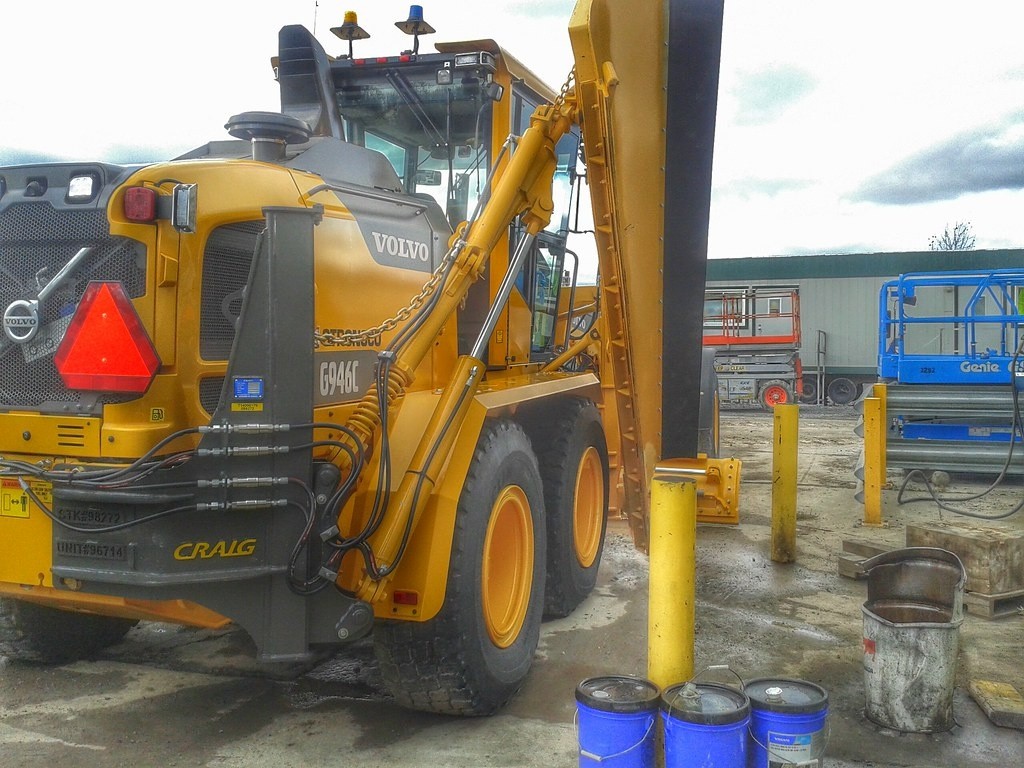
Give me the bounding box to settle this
[658,665,751,768]
[573,675,662,768]
[858,548,967,733]
[740,676,832,768]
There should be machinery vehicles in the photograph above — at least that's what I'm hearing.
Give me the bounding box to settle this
[0,0,744,720]
